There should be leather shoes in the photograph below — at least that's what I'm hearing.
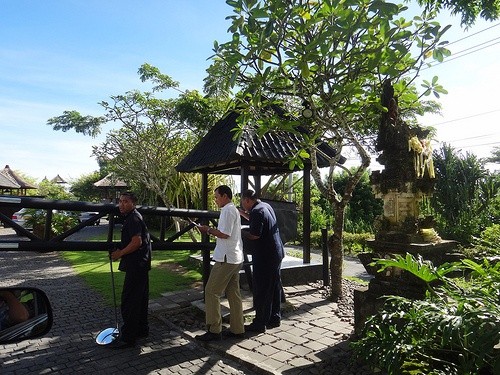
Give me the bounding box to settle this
[195,331,221,340]
[221,329,240,337]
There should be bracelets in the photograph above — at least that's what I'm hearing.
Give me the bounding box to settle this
[208,228,212,235]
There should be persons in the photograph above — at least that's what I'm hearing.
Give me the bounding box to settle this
[106,191,151,349]
[197,185,244,342]
[0,288,36,333]
[237,189,286,332]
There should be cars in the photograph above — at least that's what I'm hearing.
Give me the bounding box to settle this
[0,207,100,227]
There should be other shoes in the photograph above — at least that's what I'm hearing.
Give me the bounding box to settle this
[267,320,280,327]
[110,334,149,348]
[244,323,266,332]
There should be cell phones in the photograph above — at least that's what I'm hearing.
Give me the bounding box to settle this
[192,222,199,227]
